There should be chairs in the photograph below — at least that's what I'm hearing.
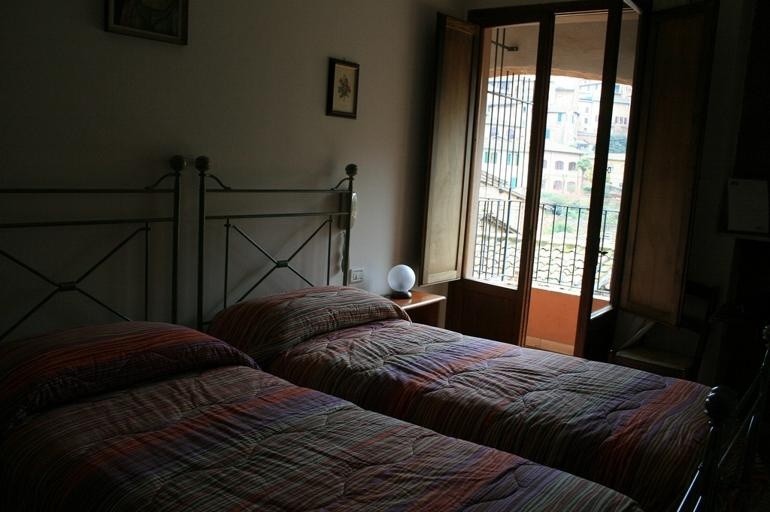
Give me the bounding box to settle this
[610,317,708,382]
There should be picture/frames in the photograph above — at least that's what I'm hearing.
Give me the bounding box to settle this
[102,1,190,48]
[324,56,360,119]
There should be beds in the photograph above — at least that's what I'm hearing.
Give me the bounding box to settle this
[190,151,769,512]
[0,152,725,512]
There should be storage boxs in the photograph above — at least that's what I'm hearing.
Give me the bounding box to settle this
[724,177,770,238]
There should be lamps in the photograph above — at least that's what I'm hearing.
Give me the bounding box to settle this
[386,263,417,301]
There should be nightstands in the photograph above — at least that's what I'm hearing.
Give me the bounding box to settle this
[374,287,448,329]
